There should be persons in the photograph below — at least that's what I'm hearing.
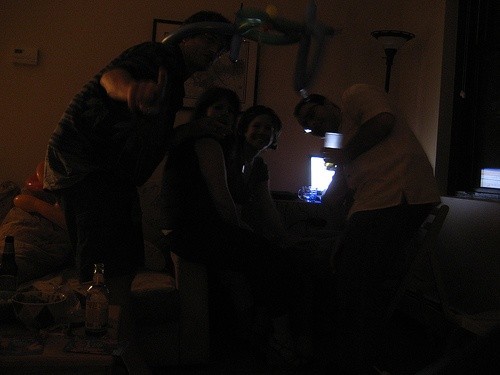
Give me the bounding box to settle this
[293,84,441,375]
[0,11,304,368]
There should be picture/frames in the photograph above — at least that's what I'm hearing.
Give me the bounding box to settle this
[152,18,262,112]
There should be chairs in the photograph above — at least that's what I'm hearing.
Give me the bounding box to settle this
[384,205,453,322]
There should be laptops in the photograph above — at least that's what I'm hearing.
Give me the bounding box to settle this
[308,154,336,196]
[476,168,500,194]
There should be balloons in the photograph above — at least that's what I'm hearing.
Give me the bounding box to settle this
[161,6,338,65]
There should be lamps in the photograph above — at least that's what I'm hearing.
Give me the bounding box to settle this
[370,30,416,94]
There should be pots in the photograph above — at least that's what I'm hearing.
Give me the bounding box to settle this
[12,290,74,325]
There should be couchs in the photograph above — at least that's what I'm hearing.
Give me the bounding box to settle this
[0,239,210,375]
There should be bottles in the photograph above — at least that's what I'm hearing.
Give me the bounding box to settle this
[0,236,19,274]
[85,264,110,337]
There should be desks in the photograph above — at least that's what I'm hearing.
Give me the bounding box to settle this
[0,304,132,375]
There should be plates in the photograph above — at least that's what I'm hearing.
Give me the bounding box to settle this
[0,289,15,311]
